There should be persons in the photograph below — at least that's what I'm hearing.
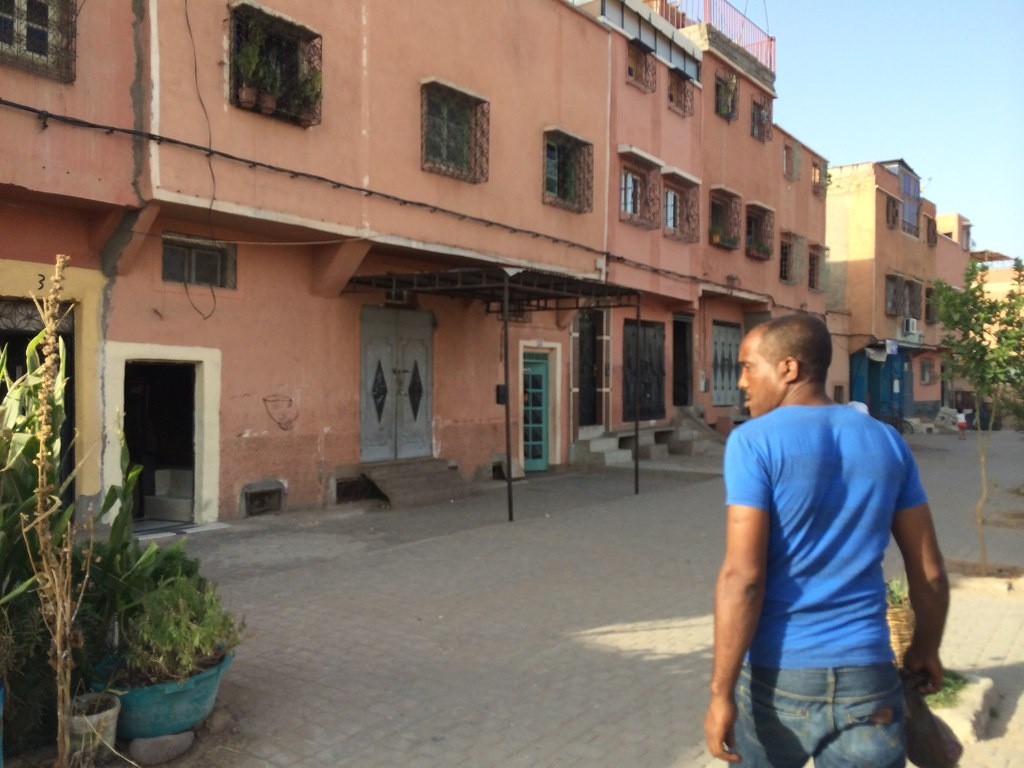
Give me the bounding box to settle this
[955,409,968,441]
[702,311,950,768]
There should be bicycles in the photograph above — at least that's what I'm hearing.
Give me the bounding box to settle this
[902,420,913,434]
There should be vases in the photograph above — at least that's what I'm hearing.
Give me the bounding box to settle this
[71,693,121,760]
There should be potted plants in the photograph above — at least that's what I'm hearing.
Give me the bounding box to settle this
[72,410,249,739]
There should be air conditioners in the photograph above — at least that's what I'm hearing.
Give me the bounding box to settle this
[904,318,916,334]
